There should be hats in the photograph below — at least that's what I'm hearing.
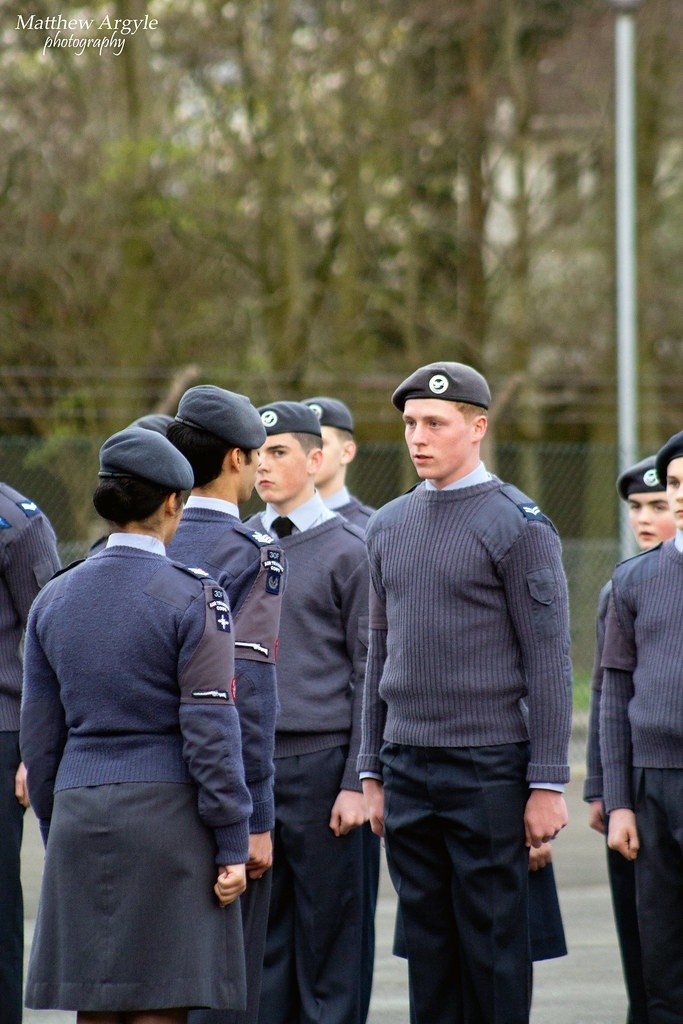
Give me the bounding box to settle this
[96,427,196,491]
[129,414,174,437]
[391,363,491,410]
[302,396,355,433]
[175,384,265,449]
[256,401,322,440]
[654,431,683,490]
[616,455,666,501]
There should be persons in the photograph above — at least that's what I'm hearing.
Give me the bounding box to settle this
[583,431,683,1024]
[241,397,380,1024]
[355,362,573,1024]
[85,385,288,1023]
[19,427,253,1024]
[0,480,62,1024]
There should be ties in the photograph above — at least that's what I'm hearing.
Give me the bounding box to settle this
[271,518,294,539]
